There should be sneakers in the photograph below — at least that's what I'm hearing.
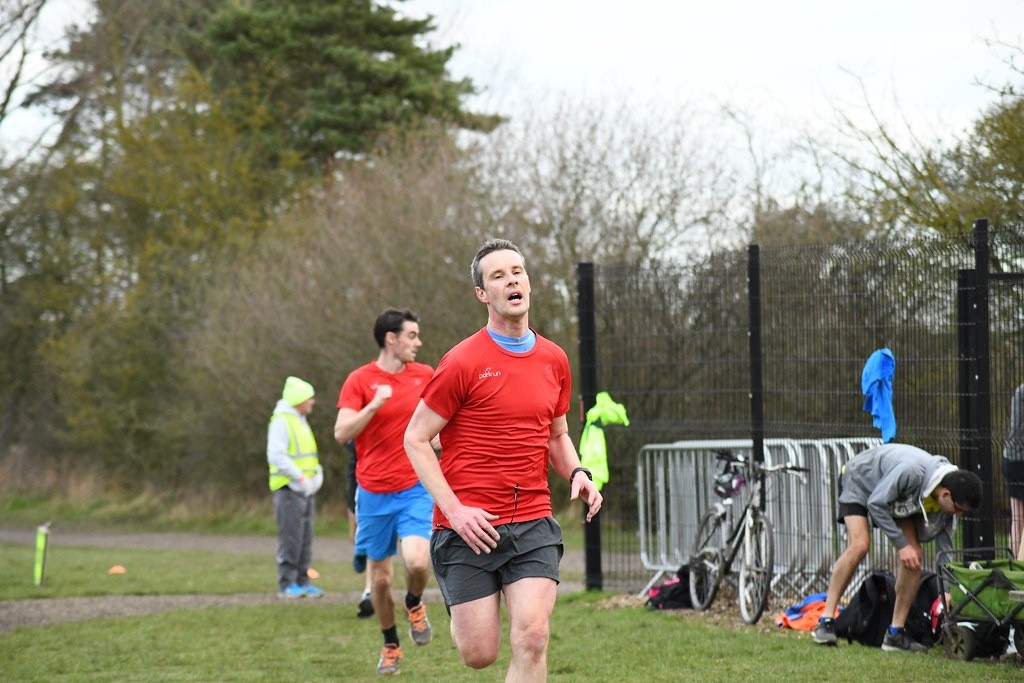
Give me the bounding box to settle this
[277,583,307,598]
[402,600,431,645]
[377,643,404,675]
[303,585,322,597]
[812,617,837,643]
[881,627,928,652]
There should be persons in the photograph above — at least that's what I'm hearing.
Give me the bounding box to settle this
[267,376,324,598]
[812,443,983,655]
[1003,385,1024,561]
[403,239,602,683]
[334,308,444,676]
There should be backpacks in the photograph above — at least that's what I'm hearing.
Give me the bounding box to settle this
[904,571,945,648]
[835,570,896,646]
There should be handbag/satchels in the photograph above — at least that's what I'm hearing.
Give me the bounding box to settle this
[644,559,708,610]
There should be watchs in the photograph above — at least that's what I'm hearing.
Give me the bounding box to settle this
[569,467,592,485]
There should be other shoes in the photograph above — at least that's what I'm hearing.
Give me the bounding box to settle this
[357,593,374,618]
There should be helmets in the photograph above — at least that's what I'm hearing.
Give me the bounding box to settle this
[713,472,743,499]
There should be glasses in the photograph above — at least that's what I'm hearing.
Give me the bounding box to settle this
[950,502,968,513]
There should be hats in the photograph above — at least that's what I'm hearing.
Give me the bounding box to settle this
[282,376,314,406]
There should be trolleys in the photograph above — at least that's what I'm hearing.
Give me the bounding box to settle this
[937,546,1024,662]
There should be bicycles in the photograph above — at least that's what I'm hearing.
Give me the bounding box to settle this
[688,445,811,625]
